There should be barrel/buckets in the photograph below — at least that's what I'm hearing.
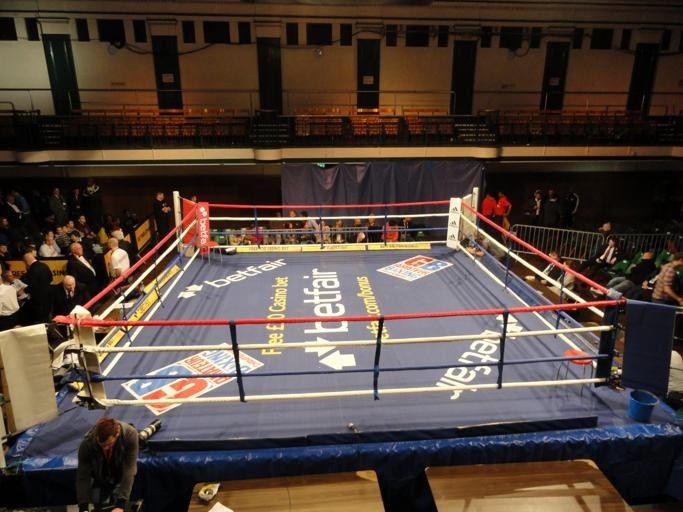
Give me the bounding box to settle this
[629,390,659,421]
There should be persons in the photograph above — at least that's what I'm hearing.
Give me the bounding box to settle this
[74,416,140,512]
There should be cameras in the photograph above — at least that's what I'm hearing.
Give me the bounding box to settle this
[138,418,162,447]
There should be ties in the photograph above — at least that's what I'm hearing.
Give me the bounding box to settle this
[603,246,613,261]
[547,263,554,272]
